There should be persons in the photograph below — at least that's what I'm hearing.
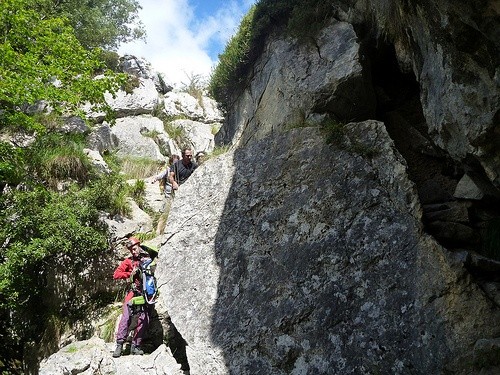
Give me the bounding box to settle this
[151,154,181,202]
[112,239,154,358]
[195,151,208,163]
[169,146,198,191]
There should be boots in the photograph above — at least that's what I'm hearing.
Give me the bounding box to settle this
[112,343,123,357]
[131,344,144,355]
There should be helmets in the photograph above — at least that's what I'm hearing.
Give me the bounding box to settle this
[126,238,140,250]
